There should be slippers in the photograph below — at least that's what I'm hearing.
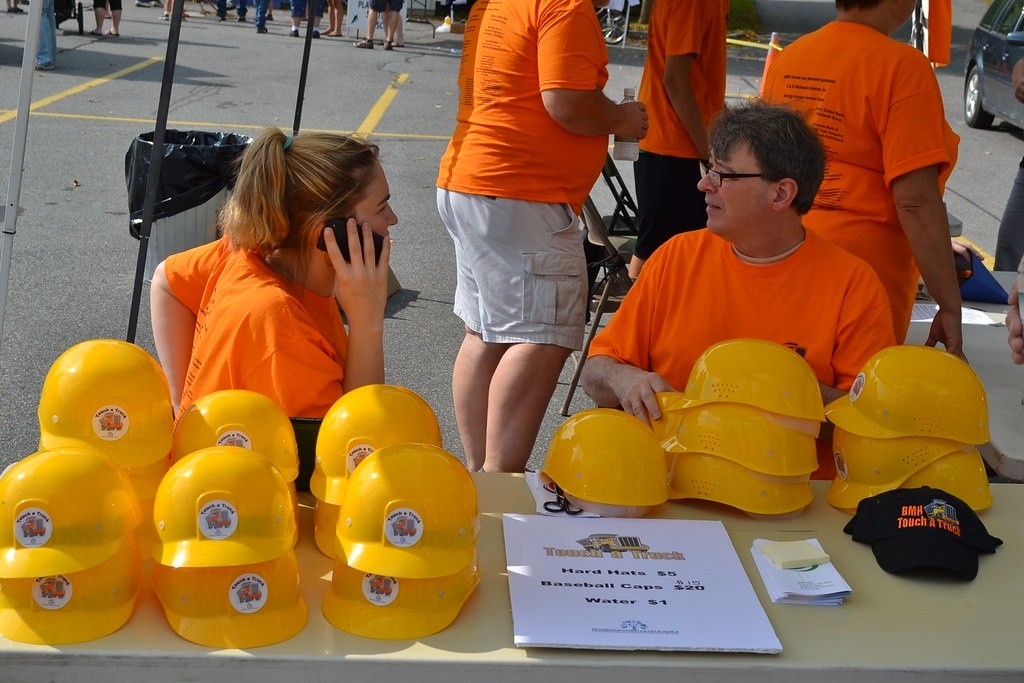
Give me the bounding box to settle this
[108,29,119,36]
[90,30,102,35]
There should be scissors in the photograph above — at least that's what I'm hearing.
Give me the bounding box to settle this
[543,480,584,514]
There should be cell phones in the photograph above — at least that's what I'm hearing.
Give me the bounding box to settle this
[315,216,383,266]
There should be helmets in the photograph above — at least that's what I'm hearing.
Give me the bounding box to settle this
[650,337,828,519]
[539,408,667,516]
[0,338,482,648]
[823,344,993,515]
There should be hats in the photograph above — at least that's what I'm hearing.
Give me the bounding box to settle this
[843,485,1003,579]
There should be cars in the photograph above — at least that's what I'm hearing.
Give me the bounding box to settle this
[962,0,1024,129]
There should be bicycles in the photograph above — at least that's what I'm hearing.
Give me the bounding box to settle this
[596,6,630,45]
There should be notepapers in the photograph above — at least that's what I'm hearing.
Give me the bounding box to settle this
[758,539,831,569]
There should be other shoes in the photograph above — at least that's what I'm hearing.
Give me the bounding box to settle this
[160,13,168,19]
[135,1,150,6]
[239,15,246,21]
[152,1,164,7]
[35,63,56,69]
[353,39,372,49]
[383,42,392,50]
[265,15,273,20]
[219,16,225,21]
[290,29,299,36]
[256,26,267,33]
[312,30,320,37]
[7,7,23,13]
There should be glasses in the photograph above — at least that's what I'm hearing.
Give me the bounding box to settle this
[699,160,764,186]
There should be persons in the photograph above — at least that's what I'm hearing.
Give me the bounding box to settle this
[88,0,122,37]
[352,0,405,50]
[34,0,57,70]
[135,0,190,22]
[628,0,728,276]
[436,0,648,476]
[216,0,274,33]
[6,0,23,13]
[760,0,1024,365]
[579,100,897,480]
[149,126,397,462]
[289,0,344,38]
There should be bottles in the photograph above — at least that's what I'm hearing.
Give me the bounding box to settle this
[612,87,640,161]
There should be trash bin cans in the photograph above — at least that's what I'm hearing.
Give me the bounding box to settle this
[134,128,256,287]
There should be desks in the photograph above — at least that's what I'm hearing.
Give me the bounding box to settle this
[903,268,1024,484]
[946,210,964,239]
[0,467,1024,683]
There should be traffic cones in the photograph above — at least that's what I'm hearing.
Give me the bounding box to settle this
[758,32,780,101]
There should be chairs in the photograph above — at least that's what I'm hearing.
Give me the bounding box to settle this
[558,193,635,420]
[599,152,638,238]
